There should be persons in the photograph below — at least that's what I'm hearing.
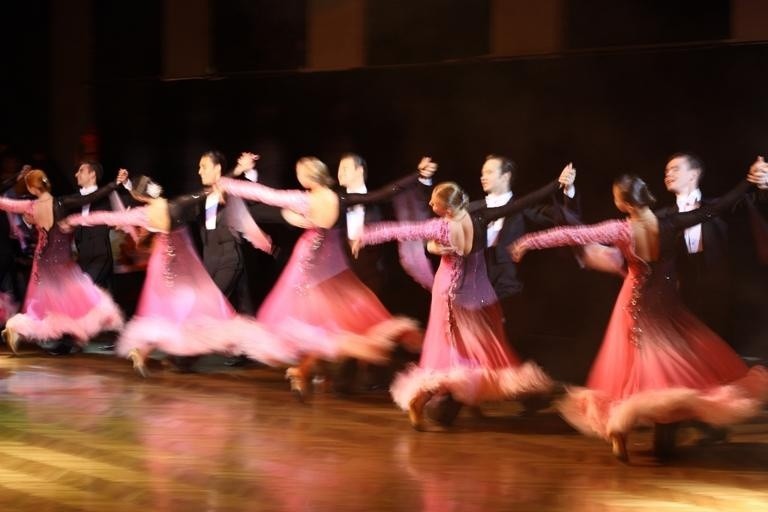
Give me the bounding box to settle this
[208,154,436,406]
[414,151,577,422]
[1,162,130,359]
[582,153,768,465]
[510,155,768,465]
[275,149,435,396]
[345,157,577,432]
[24,159,116,359]
[165,150,260,377]
[54,173,254,382]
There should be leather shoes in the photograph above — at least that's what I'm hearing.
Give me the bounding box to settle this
[608,429,635,464]
[407,398,427,431]
[0,328,23,357]
[125,350,150,379]
[283,364,312,405]
